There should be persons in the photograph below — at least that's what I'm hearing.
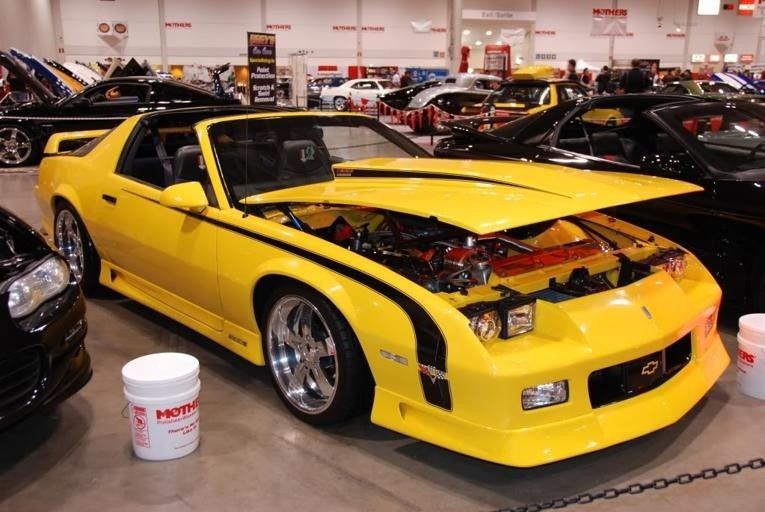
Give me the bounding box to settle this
[228,72,235,81]
[561,57,692,96]
[98,56,154,80]
[390,70,414,89]
[696,61,765,86]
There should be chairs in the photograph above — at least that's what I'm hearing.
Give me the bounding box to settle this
[559,127,659,167]
[172,138,327,207]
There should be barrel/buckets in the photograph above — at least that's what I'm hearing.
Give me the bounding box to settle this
[121,351,202,462]
[737,313,765,402]
